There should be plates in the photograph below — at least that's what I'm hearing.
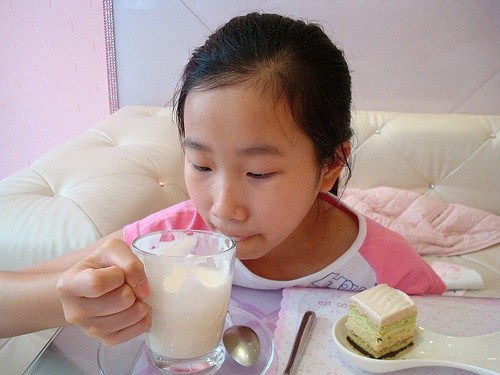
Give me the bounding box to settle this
[97,305,274,375]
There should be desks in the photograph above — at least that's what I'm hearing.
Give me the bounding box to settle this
[22,285,500,375]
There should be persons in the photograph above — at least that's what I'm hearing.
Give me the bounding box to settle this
[0,12,448,345]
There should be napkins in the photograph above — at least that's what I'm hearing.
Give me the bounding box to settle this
[273,288,500,375]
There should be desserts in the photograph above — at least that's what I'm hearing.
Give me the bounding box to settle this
[346,282,418,361]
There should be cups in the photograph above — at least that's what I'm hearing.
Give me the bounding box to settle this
[130,229,237,375]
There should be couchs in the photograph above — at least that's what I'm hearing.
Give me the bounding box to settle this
[0,106,500,298]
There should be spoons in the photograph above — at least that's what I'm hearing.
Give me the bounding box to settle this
[223,311,260,367]
